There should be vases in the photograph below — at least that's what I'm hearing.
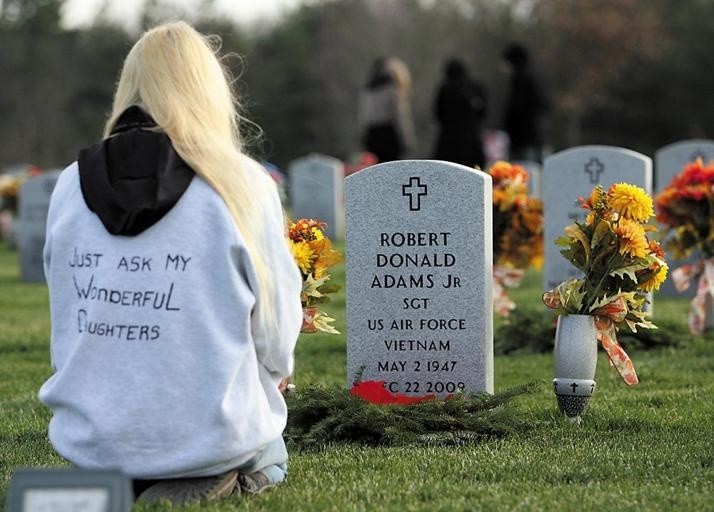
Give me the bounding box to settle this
[551,311,599,422]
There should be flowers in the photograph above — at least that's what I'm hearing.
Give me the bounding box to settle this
[488,162,550,315]
[652,157,714,329]
[541,177,669,387]
[282,217,343,338]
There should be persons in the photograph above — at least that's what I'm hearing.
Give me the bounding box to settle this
[500,39,568,164]
[360,56,415,163]
[33,16,304,508]
[431,56,488,170]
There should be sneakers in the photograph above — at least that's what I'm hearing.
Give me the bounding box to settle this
[134,469,240,512]
